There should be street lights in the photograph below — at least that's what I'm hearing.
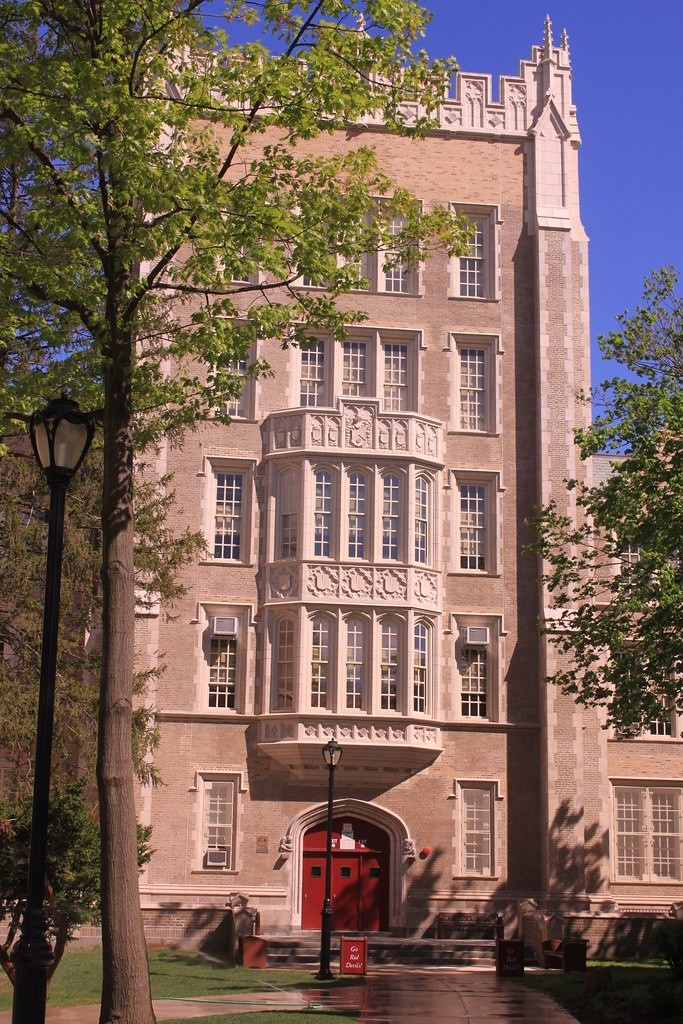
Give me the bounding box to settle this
[13,389,98,1024]
[315,737,340,982]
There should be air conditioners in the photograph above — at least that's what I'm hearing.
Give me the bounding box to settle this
[213,616,238,635]
[206,850,227,866]
[464,627,489,645]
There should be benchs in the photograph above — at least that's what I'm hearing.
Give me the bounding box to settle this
[434,912,506,939]
[235,933,267,969]
[539,938,590,971]
